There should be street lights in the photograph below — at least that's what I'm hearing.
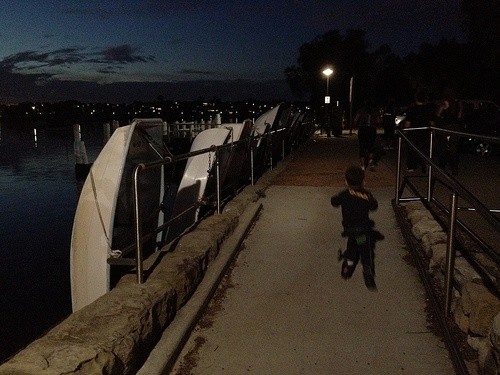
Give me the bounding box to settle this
[322,66,334,134]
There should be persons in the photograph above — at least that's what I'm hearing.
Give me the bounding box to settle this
[358,113,376,171]
[404,91,430,173]
[442,106,464,177]
[331,166,378,292]
[383,101,395,147]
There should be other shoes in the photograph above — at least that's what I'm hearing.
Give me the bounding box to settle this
[364,273,376,291]
[406,169,415,173]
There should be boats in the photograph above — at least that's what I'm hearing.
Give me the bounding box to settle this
[217,118,255,200]
[69,116,165,315]
[253,104,316,161]
[164,127,233,247]
